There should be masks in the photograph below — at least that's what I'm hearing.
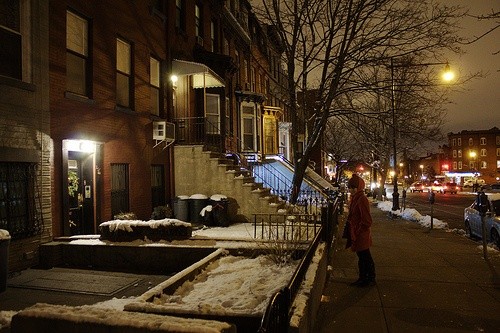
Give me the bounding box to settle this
[348,188,357,195]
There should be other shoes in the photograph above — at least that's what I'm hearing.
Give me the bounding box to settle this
[349,274,377,288]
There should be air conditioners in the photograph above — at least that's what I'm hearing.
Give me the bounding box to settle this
[153,121,176,144]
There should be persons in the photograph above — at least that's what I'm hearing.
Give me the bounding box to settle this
[344,174,377,287]
[428,191,435,204]
[372,184,379,202]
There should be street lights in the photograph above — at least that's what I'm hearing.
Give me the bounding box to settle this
[389,59,451,212]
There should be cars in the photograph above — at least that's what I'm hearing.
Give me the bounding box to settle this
[464,192,500,245]
[335,169,500,203]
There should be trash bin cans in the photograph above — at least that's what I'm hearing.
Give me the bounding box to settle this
[172,195,189,222]
[0,229,12,292]
[189,193,208,226]
[210,194,228,227]
